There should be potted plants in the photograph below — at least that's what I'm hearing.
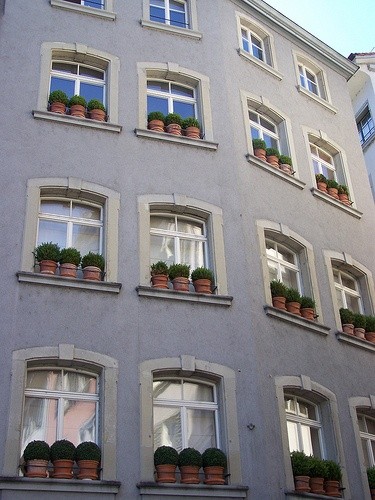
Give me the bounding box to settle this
[290,452,341,498]
[49,90,106,122]
[23,440,99,480]
[152,444,227,485]
[149,262,214,296]
[148,112,202,139]
[366,467,375,500]
[339,309,375,344]
[252,138,292,174]
[269,280,315,320]
[316,173,349,204]
[32,241,105,282]
